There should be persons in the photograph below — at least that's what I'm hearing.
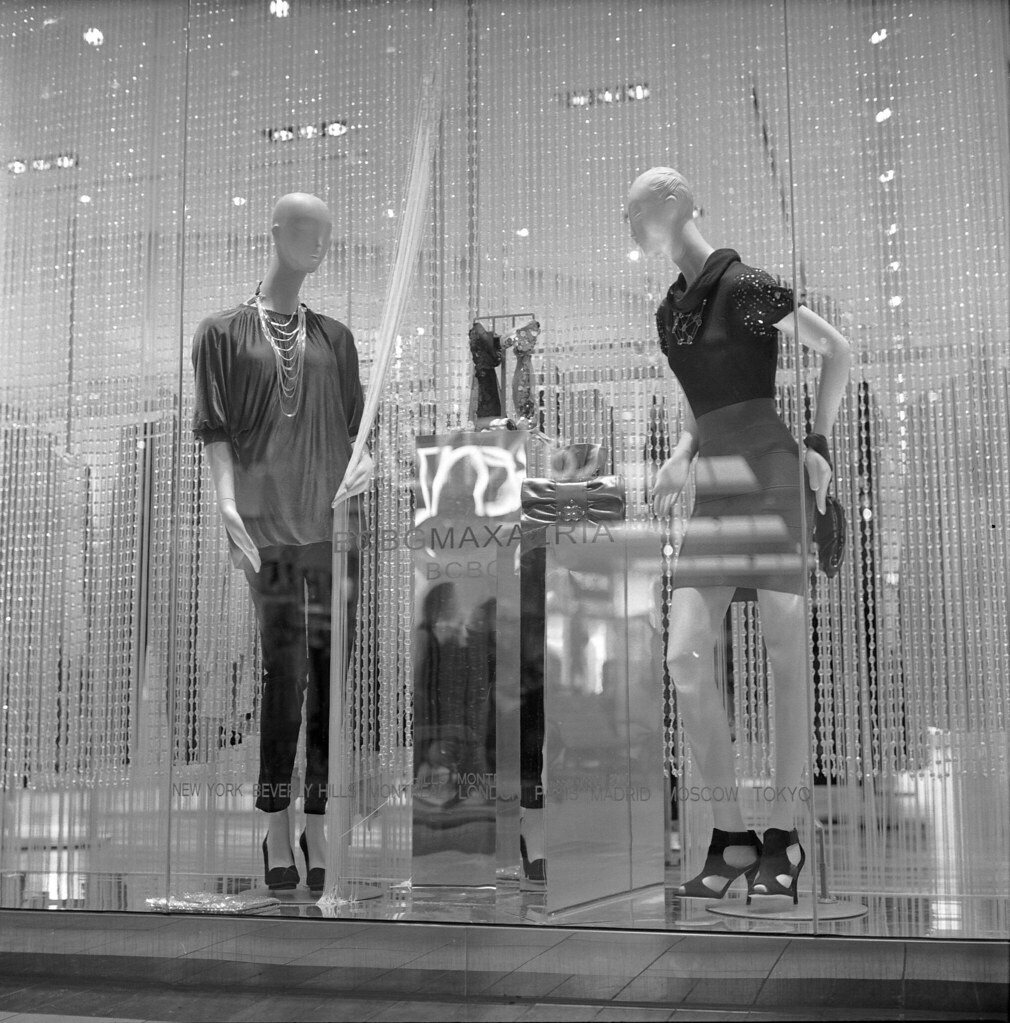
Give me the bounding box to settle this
[627,166,853,907]
[190,192,374,890]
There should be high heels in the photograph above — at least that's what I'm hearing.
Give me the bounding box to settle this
[747,828,805,904]
[673,828,763,905]
[519,817,546,880]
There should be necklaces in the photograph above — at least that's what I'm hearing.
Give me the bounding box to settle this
[255,294,307,418]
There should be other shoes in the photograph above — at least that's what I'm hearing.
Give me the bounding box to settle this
[299,827,325,889]
[262,830,299,887]
